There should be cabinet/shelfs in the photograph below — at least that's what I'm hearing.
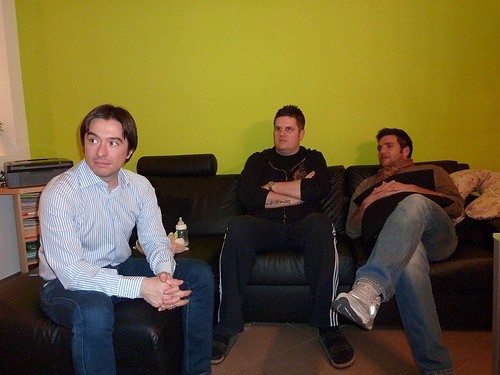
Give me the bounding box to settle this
[0,186,46,273]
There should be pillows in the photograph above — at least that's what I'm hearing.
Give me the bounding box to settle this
[130,196,193,244]
[353,169,455,246]
[449,169,500,225]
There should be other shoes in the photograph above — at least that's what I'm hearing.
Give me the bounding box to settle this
[318,328,357,368]
[212,326,237,363]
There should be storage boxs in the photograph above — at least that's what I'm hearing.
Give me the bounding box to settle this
[3,158,74,188]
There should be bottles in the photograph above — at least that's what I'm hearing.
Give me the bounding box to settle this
[175,216,189,247]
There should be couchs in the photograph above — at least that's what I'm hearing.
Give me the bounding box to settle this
[0,154,500,375]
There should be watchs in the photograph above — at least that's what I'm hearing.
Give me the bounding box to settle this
[267,181,274,190]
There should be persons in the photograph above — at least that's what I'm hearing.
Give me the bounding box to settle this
[335,127,464,375]
[209,105,355,367]
[38,104,214,375]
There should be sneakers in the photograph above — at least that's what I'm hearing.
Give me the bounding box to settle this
[333,283,383,332]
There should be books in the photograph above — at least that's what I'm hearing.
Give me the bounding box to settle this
[21,192,39,229]
